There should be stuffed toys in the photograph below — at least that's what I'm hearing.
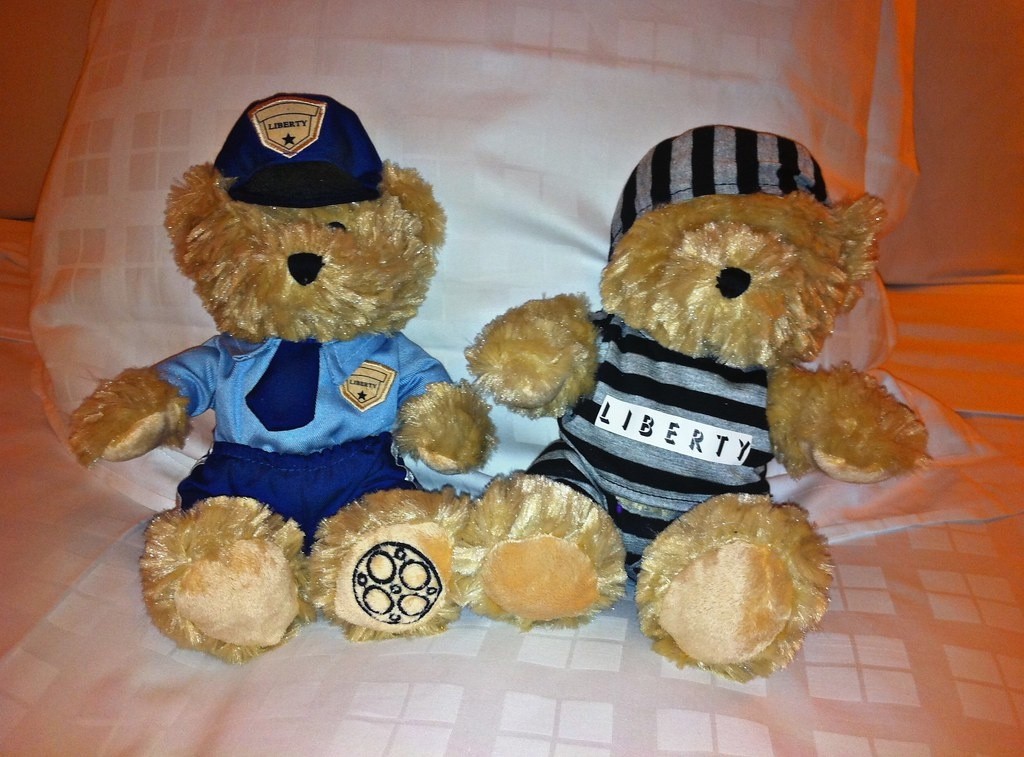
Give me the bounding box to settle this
[72,93,501,665]
[447,123,932,684]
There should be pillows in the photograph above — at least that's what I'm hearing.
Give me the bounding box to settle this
[30,0,925,515]
[872,0,1024,287]
